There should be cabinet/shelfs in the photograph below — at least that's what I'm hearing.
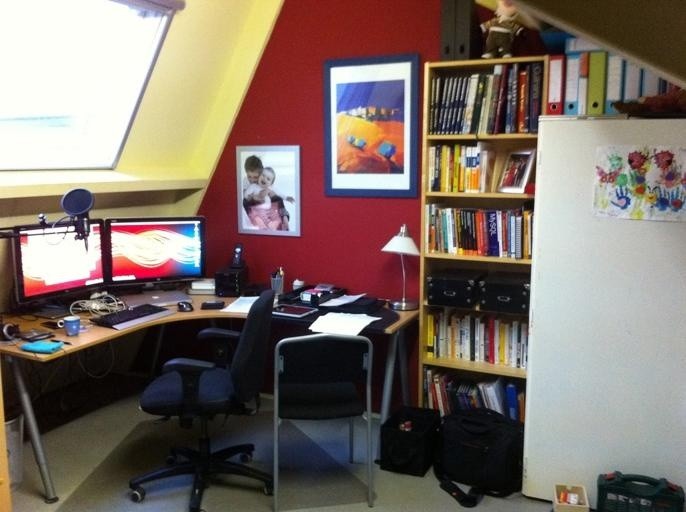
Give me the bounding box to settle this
[419,56,549,422]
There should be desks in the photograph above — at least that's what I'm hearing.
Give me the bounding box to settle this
[0,289,419,505]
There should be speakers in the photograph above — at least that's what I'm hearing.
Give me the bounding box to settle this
[215,268,244,297]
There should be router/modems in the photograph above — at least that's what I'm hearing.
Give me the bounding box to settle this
[191,278,215,290]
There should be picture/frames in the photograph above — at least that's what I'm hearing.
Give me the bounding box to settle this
[496,149,536,193]
[236,144,301,237]
[322,52,418,197]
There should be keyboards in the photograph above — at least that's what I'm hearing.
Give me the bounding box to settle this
[32,308,81,318]
[90,303,176,332]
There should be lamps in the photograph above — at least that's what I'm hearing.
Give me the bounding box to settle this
[380,224,420,311]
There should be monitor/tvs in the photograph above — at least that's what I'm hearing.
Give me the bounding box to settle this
[12,219,105,318]
[105,215,207,292]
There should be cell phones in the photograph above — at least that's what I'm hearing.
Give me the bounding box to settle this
[202,301,224,308]
[40,321,62,329]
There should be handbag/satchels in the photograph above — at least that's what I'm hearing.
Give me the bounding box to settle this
[434,407,524,499]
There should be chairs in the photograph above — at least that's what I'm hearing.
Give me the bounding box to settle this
[128,289,275,511]
[273,333,373,512]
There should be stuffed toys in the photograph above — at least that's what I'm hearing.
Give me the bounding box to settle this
[472,0,532,60]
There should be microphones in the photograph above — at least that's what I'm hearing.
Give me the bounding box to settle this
[61,187,95,239]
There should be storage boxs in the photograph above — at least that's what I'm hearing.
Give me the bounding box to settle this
[552,483,589,512]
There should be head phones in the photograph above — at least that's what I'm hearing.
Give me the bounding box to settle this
[0,323,20,340]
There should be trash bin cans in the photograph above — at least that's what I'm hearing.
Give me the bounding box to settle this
[5,408,25,493]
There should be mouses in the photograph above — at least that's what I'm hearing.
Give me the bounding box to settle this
[177,301,193,311]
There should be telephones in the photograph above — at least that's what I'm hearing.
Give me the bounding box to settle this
[230,243,246,269]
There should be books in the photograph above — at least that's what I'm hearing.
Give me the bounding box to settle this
[423,305,528,424]
[424,200,533,262]
[428,139,496,195]
[430,64,542,136]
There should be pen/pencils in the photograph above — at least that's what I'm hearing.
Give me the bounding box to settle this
[30,334,54,342]
[51,340,72,345]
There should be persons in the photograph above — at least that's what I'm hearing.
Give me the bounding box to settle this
[243,154,295,231]
[502,160,517,187]
[514,157,527,187]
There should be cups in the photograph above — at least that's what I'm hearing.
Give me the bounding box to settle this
[57,315,81,337]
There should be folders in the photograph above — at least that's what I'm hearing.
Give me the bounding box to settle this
[546,36,666,116]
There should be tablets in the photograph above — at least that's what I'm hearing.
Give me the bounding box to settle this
[272,302,319,318]
